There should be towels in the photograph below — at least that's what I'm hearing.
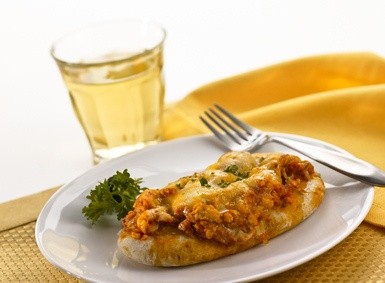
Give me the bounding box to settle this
[162,53,385,229]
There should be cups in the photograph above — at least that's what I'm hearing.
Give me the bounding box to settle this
[50,26,165,164]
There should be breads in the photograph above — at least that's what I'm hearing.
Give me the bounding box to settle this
[117,150,326,268]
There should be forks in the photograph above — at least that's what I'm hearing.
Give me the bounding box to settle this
[199,104,385,189]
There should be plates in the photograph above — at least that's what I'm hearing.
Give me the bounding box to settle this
[35,131,374,278]
